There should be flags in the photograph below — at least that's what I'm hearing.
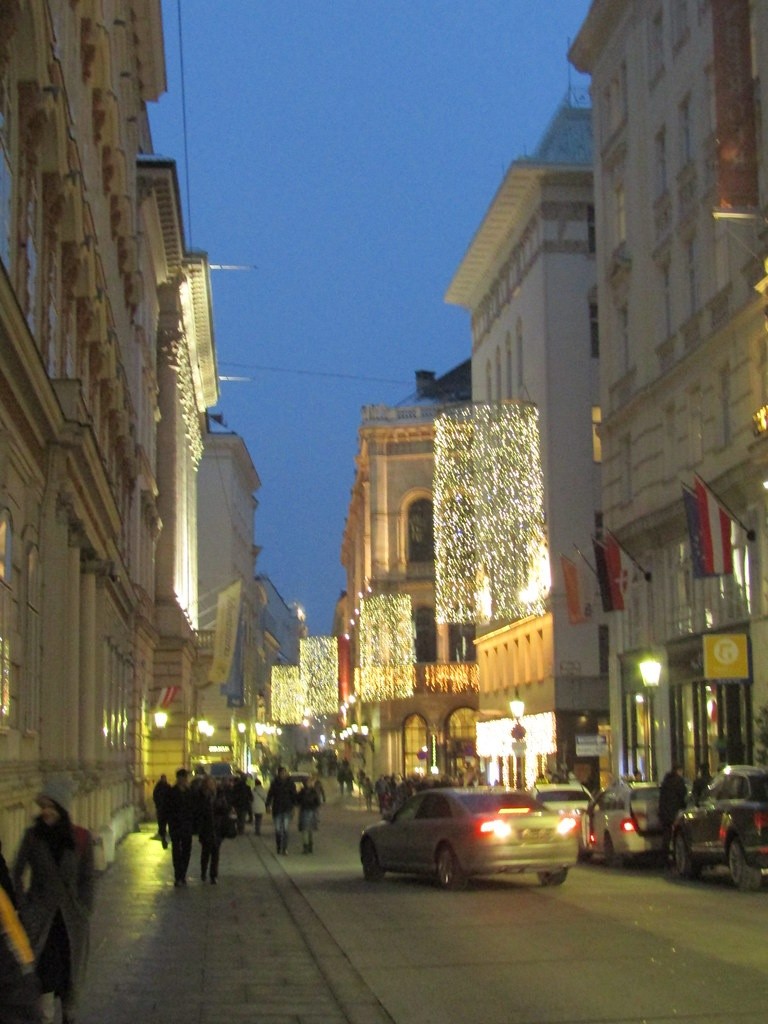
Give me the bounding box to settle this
[681,479,732,578]
[156,686,176,710]
[560,536,633,626]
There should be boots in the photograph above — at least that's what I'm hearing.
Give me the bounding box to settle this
[60,991,78,1024]
[38,991,56,1024]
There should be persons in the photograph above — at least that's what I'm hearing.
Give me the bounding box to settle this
[534,763,578,784]
[691,763,714,807]
[0,777,92,1024]
[633,769,642,782]
[154,763,264,887]
[266,767,326,856]
[317,749,354,794]
[657,761,687,867]
[357,768,499,821]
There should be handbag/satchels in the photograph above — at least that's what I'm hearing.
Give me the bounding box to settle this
[224,807,241,839]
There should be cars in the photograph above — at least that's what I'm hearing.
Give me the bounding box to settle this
[583,782,666,868]
[531,783,600,815]
[674,767,768,893]
[290,771,312,793]
[359,787,581,893]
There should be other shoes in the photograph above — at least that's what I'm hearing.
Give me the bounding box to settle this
[175,876,187,887]
[246,820,253,824]
[303,843,315,854]
[277,846,289,856]
[201,876,217,885]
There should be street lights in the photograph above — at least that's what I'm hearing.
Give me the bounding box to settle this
[508,688,525,790]
[640,659,662,784]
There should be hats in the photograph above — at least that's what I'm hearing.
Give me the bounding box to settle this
[39,780,72,812]
